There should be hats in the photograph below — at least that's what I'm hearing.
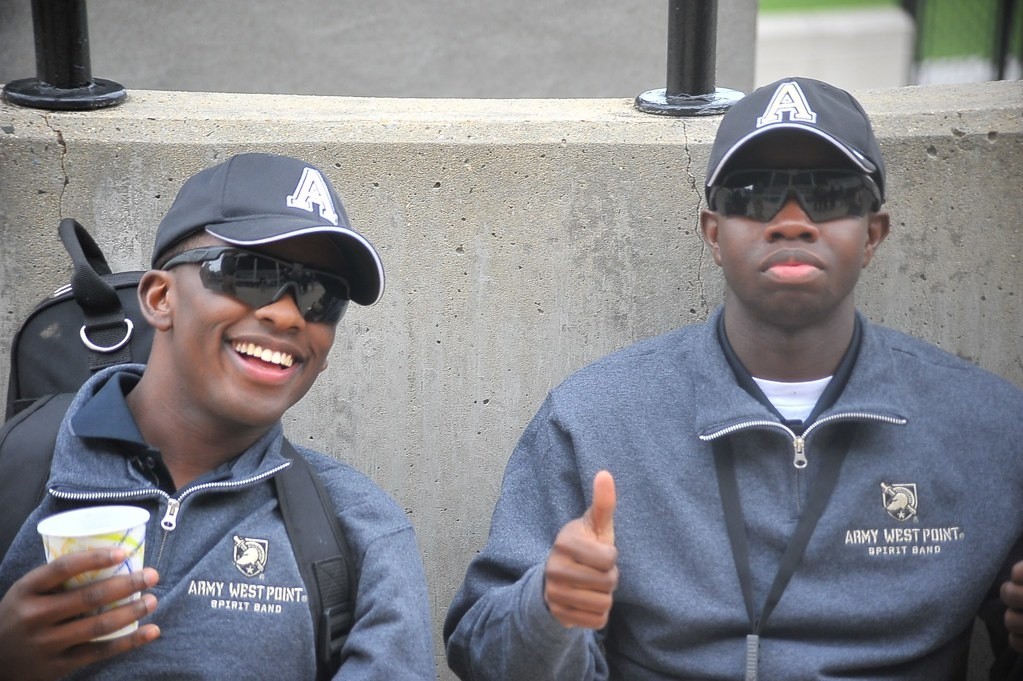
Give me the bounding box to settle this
[704,77,886,213]
[153,153,386,307]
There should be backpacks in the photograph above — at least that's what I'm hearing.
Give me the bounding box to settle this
[1,218,357,677]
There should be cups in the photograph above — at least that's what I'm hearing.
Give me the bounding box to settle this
[37,505,150,642]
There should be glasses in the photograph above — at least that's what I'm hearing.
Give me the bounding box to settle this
[709,167,881,223]
[160,246,351,325]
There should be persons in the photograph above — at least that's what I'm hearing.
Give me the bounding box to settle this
[442,75,1023,681]
[0,153,435,680]
[812,184,845,210]
